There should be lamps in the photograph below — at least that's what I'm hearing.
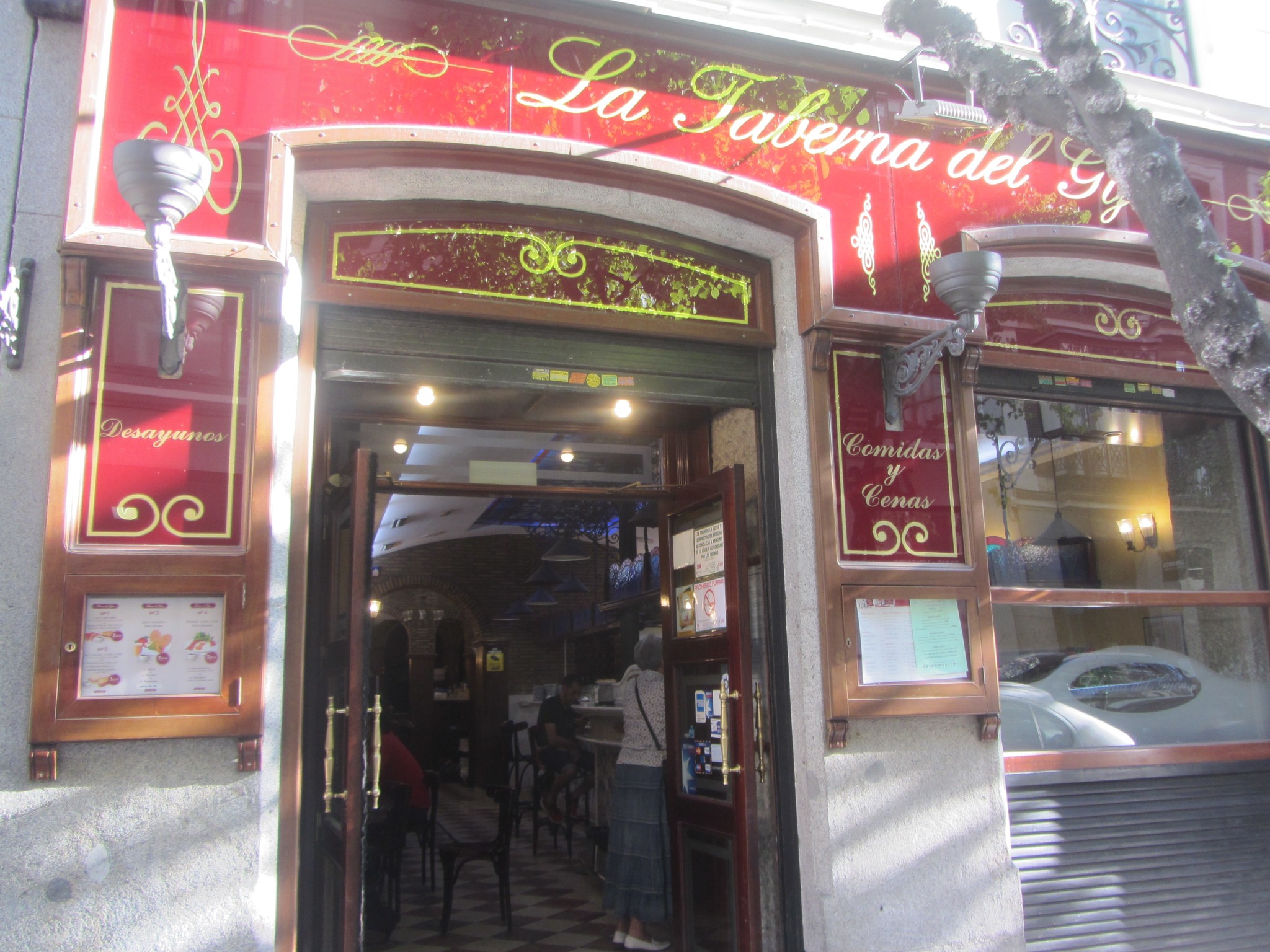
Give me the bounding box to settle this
[525,586,558,605]
[493,606,521,621]
[560,447,575,462]
[877,251,1002,425]
[1116,513,1158,552]
[393,438,408,454]
[554,562,587,593]
[1031,440,1093,546]
[112,139,212,380]
[541,527,591,561]
[886,45,989,130]
[524,560,561,586]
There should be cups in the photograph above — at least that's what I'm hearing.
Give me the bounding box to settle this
[1058,536,1101,590]
[544,683,556,698]
[533,685,546,702]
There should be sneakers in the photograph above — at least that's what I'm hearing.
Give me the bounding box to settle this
[539,797,565,824]
[561,797,578,818]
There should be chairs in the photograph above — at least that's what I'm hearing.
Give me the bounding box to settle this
[364,713,592,945]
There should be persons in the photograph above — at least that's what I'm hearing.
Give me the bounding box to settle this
[608,634,673,950]
[369,716,430,823]
[535,674,590,824]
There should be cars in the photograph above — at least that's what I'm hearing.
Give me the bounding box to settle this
[994,644,1268,752]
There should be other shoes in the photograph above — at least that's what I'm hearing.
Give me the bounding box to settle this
[612,930,671,952]
